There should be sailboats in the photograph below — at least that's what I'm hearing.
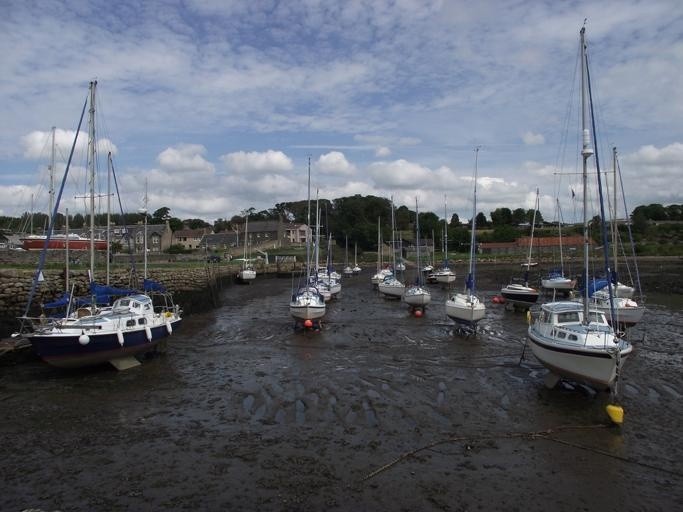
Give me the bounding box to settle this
[236,215,256,280]
[288,155,361,320]
[370,150,485,322]
